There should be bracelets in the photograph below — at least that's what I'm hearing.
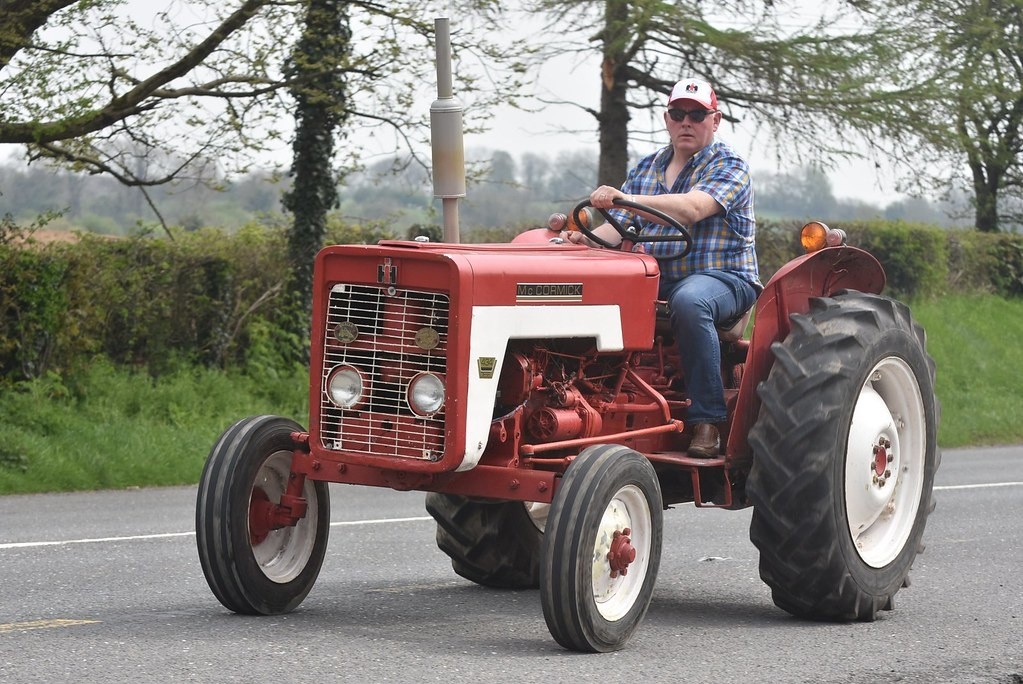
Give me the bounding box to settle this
[632,196,635,203]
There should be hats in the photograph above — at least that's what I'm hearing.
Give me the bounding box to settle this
[667,78,717,111]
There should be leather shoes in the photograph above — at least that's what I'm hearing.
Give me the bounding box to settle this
[686,422,721,459]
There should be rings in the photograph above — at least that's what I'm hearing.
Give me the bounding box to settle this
[601,194,605,196]
[562,231,567,234]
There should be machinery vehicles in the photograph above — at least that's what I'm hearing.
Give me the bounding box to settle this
[195,20,941,654]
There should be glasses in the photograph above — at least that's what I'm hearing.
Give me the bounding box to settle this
[667,108,716,122]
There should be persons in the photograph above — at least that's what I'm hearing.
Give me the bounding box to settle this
[559,78,764,457]
